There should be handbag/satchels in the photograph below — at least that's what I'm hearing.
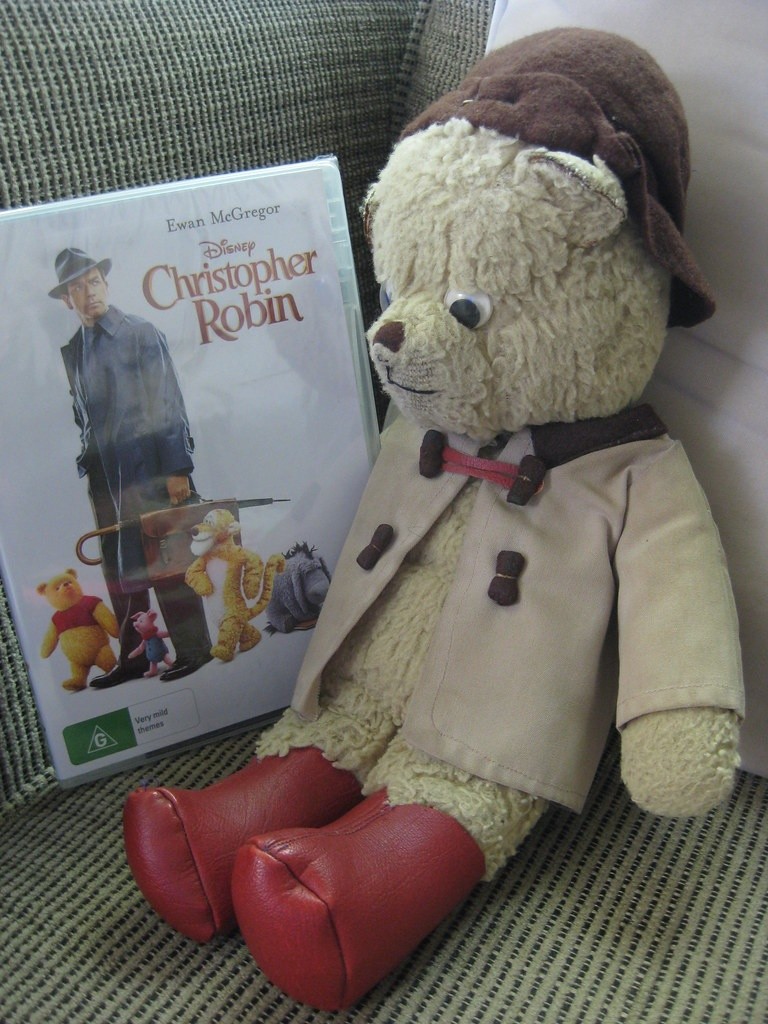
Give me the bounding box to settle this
[140,490,240,586]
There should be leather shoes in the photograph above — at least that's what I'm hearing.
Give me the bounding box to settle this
[90,665,150,689]
[160,655,215,681]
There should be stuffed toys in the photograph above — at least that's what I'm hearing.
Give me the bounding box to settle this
[128,609,174,677]
[185,508,284,661]
[122,30,746,1013]
[36,569,120,690]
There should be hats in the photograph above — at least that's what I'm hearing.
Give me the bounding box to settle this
[48,247,112,300]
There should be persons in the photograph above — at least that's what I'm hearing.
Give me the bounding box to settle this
[47,248,214,689]
